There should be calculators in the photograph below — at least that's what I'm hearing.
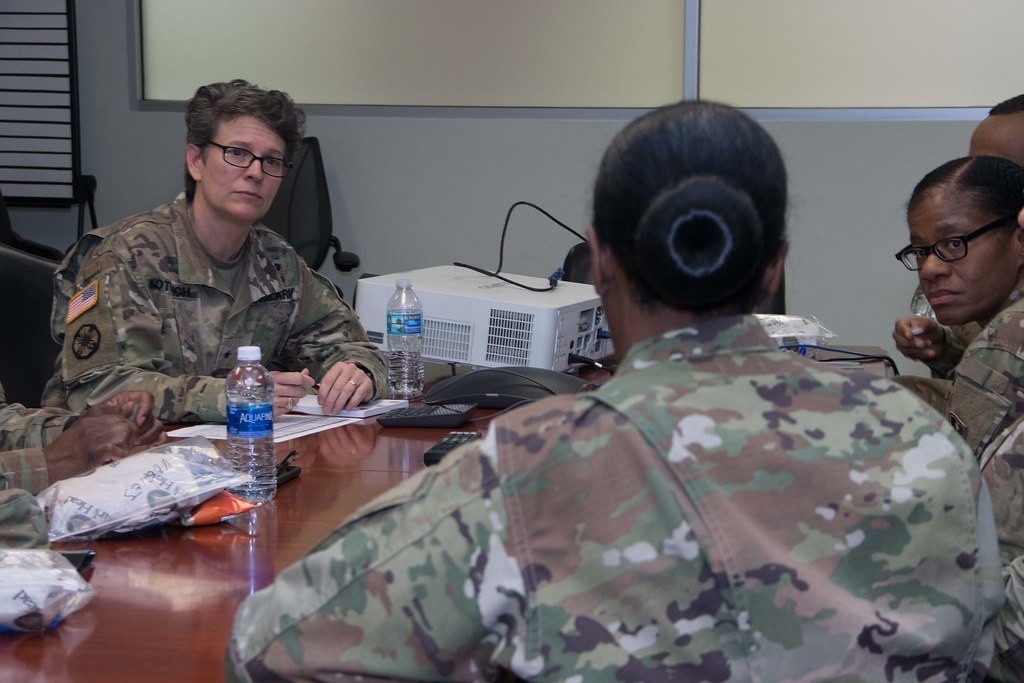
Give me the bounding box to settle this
[376,403,479,428]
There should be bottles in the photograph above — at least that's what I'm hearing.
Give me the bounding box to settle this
[910,284,937,321]
[225,347,276,500]
[387,280,424,393]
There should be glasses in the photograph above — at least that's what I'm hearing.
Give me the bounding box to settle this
[895,212,1019,270]
[200,139,293,177]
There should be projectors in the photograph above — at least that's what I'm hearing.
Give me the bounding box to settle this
[354,265,614,372]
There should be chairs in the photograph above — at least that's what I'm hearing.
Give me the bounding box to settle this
[0,243,64,412]
[252,135,360,301]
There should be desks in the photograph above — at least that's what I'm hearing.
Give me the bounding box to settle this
[0,348,889,682]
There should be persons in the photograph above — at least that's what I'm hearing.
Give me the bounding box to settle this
[41,78,390,422]
[229,100,1003,683]
[0,383,168,496]
[892,94,1024,377]
[896,155,1024,465]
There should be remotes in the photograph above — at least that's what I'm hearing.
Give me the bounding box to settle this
[424,431,482,467]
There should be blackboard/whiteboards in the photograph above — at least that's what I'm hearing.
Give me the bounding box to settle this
[126,0,1024,122]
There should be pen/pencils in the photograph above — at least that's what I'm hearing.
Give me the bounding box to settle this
[272,359,320,390]
[130,400,140,422]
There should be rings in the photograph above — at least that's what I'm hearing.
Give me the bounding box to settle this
[348,381,356,385]
[288,398,293,408]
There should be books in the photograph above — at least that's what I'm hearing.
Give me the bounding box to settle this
[291,394,409,417]
